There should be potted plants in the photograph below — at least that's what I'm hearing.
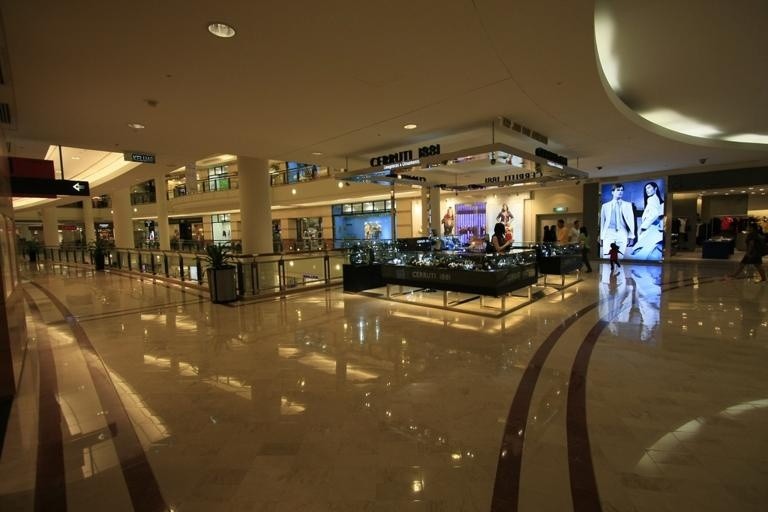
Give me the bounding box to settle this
[193,241,241,305]
[24,240,41,262]
[89,239,107,271]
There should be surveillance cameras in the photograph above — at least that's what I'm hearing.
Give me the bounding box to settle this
[699,157,706,164]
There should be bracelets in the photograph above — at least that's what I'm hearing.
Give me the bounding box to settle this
[508,240,511,244]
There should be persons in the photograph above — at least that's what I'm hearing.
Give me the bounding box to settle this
[598,182,636,259]
[363,220,382,240]
[604,243,624,270]
[495,203,514,225]
[505,223,512,248]
[490,222,515,252]
[304,228,314,246]
[442,206,455,236]
[598,262,634,335]
[149,227,154,243]
[631,181,665,260]
[311,164,317,180]
[727,223,766,283]
[542,219,593,273]
[628,267,661,342]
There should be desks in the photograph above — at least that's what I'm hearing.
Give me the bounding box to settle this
[702,238,735,259]
